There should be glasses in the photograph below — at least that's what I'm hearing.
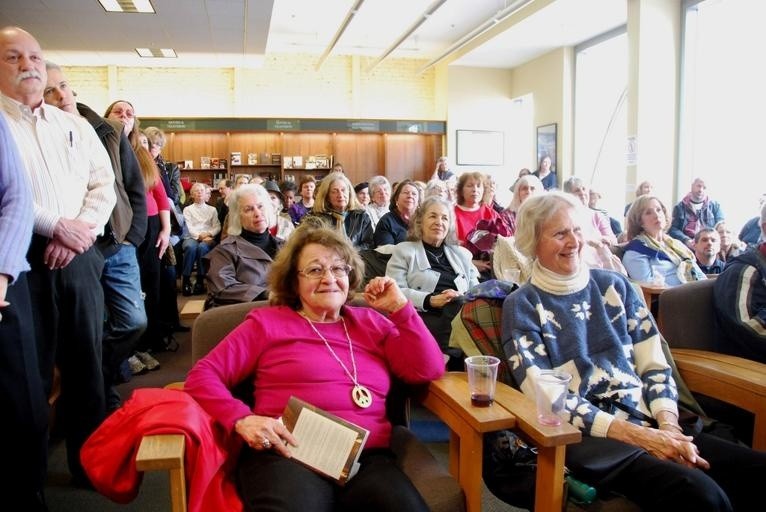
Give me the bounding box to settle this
[296,262,352,280]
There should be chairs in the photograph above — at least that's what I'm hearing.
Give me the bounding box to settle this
[135,286,765,511]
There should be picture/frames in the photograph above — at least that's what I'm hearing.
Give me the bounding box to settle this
[534,123,558,177]
[453,128,507,169]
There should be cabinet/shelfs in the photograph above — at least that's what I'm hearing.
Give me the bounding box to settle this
[163,152,334,191]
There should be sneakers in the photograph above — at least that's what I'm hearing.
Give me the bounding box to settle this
[182,280,194,296]
[107,396,122,413]
[71,471,97,492]
[173,321,191,332]
[193,283,205,295]
[127,354,146,376]
[135,350,160,371]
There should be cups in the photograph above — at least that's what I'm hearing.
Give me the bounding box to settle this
[464,354,501,408]
[650,264,669,288]
[531,368,573,428]
[503,268,521,284]
